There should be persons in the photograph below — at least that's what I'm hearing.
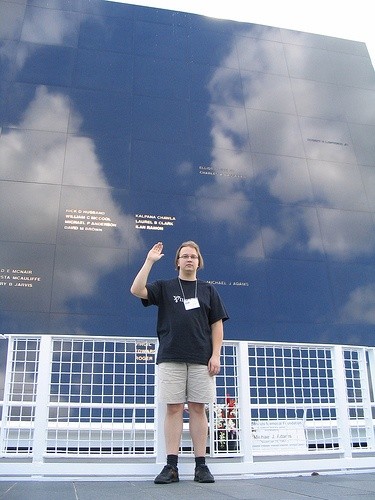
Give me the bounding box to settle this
[129,239,230,485]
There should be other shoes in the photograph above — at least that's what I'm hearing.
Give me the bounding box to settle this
[154,465,180,484]
[193,464,215,484]
